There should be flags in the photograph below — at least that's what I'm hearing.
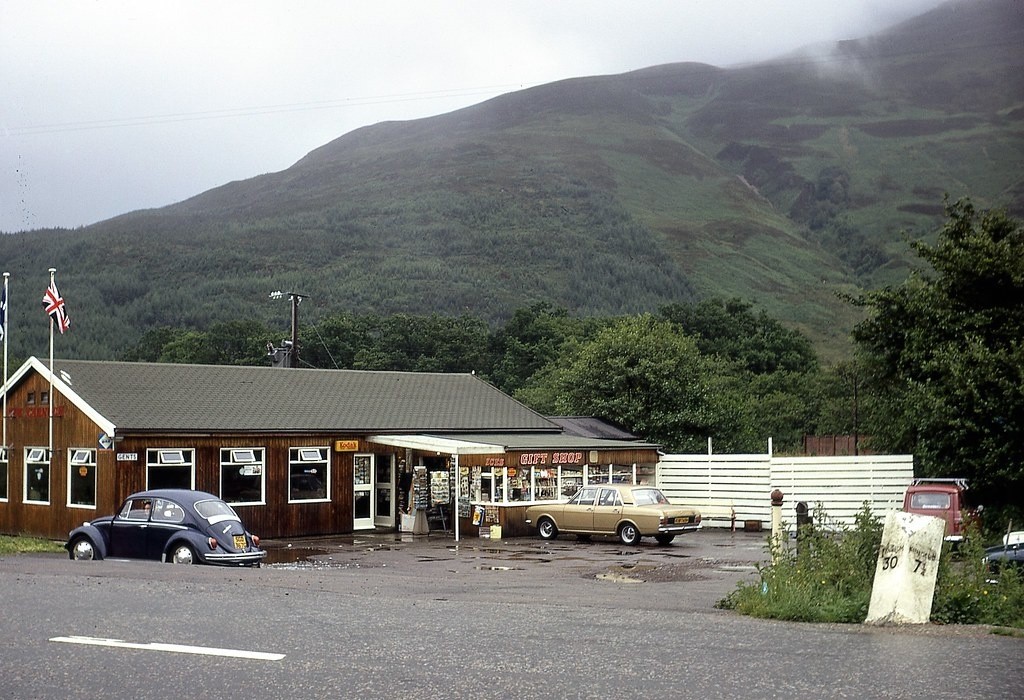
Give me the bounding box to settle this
[41,279,72,334]
[0,289,6,339]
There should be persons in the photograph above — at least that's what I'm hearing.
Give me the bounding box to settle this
[143,502,153,521]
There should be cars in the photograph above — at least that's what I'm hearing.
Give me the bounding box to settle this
[525,482,702,549]
[901,476,983,550]
[985,541,1024,573]
[64,487,267,568]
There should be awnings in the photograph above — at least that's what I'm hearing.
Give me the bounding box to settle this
[363,433,506,456]
